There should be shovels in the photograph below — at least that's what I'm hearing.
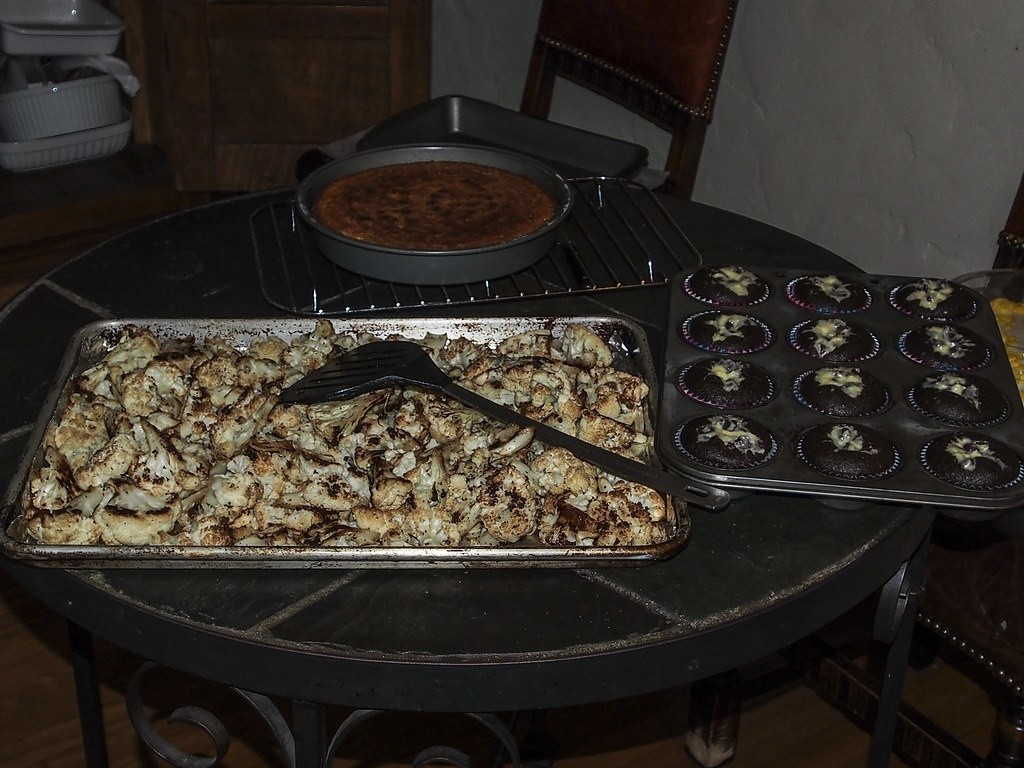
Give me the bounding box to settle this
[279,340,730,514]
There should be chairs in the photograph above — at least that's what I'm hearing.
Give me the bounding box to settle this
[684,174,1024,768]
[520,0,739,198]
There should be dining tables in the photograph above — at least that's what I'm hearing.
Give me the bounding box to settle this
[0,183,941,768]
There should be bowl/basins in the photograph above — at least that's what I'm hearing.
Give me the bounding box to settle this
[296,143,574,285]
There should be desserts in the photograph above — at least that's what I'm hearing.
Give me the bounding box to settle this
[677,264,1024,491]
